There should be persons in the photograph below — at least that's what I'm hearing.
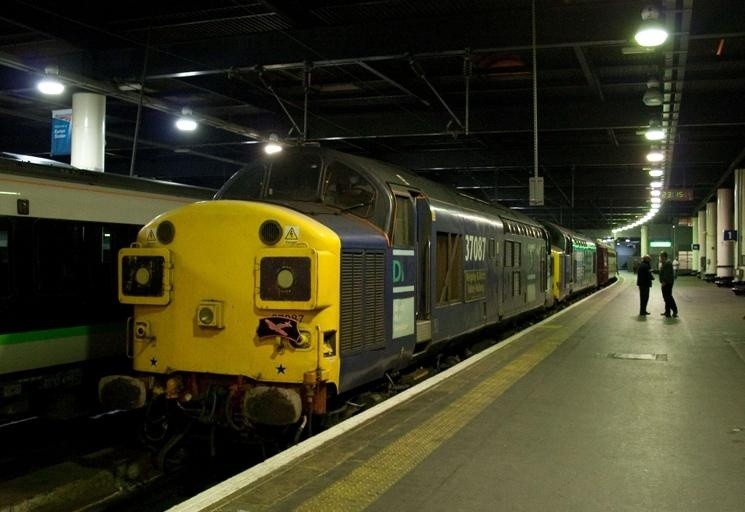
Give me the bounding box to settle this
[648,250,678,317]
[672,257,679,280]
[637,255,654,315]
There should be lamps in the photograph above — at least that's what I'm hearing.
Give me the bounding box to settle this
[642,76,691,106]
[633,3,693,47]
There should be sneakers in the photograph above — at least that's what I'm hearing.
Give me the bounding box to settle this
[640,311,650,315]
[661,311,678,317]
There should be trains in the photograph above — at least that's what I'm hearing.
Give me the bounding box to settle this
[0,149,214,400]
[99,142,617,453]
